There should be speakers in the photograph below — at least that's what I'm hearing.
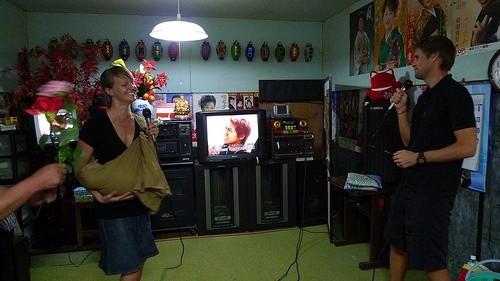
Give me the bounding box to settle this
[296,160,327,227]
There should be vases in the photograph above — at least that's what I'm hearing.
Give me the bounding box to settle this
[132,97,153,121]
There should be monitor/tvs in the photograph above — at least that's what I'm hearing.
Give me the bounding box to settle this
[196,109,267,163]
[413,80,494,194]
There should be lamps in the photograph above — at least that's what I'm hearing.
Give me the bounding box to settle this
[149,0,208,42]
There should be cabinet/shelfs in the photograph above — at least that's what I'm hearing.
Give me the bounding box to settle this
[0,131,78,247]
[150,157,328,239]
[362,99,402,180]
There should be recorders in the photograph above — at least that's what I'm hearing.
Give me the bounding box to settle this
[154,120,192,160]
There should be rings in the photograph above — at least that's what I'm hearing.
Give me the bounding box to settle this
[156,127,158,130]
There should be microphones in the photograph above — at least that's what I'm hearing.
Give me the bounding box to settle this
[142,108,157,145]
[385,79,412,118]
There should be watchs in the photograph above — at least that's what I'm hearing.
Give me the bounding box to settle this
[417,152,426,164]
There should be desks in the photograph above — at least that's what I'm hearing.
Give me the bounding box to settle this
[330,175,397,270]
[72,201,102,248]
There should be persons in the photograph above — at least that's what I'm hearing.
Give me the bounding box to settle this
[0,163,67,281]
[390,35,477,281]
[229,96,255,109]
[217,118,251,155]
[195,95,216,132]
[74,66,172,281]
[353,0,500,75]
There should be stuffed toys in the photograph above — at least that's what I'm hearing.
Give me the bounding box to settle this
[366,68,402,102]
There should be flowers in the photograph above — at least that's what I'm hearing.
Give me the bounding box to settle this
[4,32,103,127]
[23,80,87,230]
[134,58,168,99]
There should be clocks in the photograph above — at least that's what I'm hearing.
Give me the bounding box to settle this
[488,49,500,94]
